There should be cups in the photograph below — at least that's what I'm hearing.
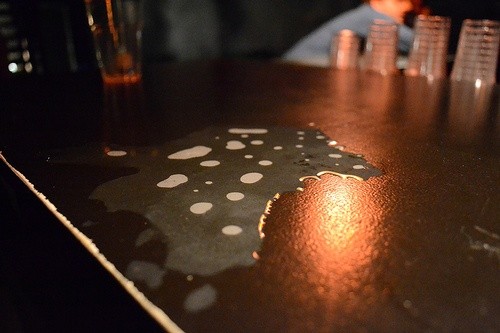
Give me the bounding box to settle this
[84,0,144,85]
[359,19,400,75]
[403,14,451,77]
[329,27,360,72]
[451,18,500,84]
